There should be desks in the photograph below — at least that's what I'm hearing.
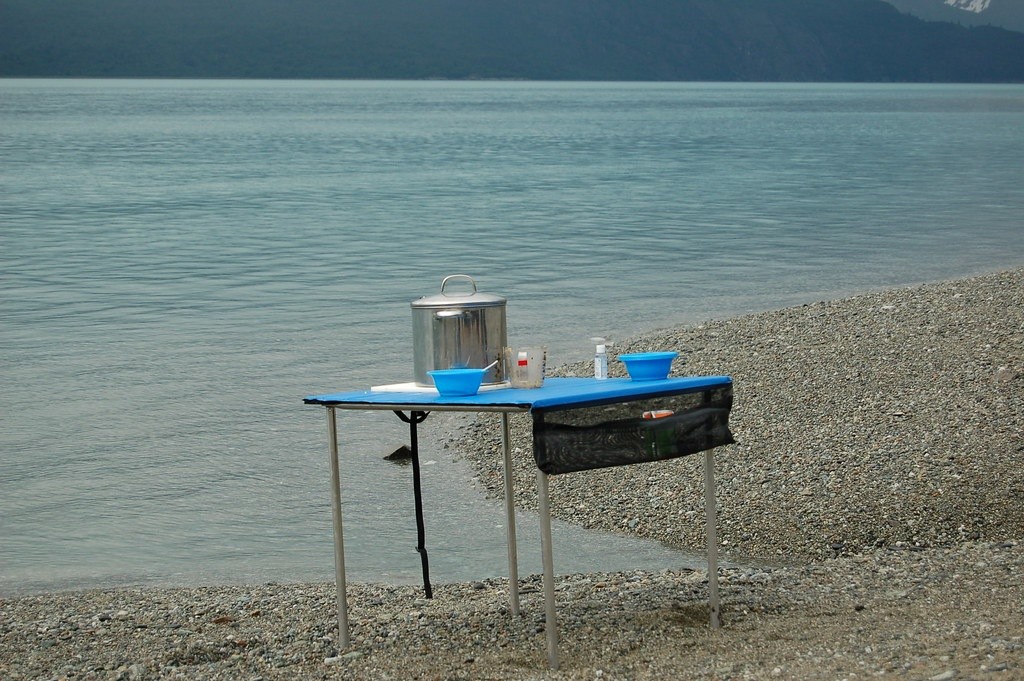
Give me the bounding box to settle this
[302,375,738,671]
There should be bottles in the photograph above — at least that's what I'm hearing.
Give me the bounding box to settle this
[594,345,608,379]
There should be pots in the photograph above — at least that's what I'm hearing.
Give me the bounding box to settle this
[410,275,508,382]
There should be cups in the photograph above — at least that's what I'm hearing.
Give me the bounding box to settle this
[507,344,548,387]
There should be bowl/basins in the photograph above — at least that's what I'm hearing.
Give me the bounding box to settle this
[425,369,490,397]
[621,352,678,379]
